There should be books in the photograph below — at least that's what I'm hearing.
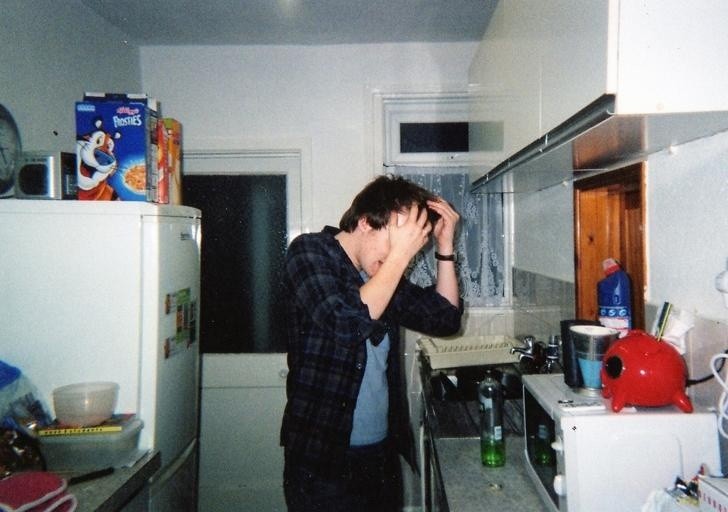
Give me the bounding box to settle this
[37,412,138,436]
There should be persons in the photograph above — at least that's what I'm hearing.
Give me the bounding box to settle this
[275,173,462,512]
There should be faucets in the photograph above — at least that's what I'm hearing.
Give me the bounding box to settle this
[510,335,547,368]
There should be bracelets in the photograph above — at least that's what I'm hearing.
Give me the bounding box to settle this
[432,251,459,264]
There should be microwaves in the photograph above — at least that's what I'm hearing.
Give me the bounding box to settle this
[522,373,723,512]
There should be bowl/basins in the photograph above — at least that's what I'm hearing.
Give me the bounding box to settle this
[54,381,117,429]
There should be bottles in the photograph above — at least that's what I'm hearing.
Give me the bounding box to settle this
[481,367,507,468]
[533,424,552,484]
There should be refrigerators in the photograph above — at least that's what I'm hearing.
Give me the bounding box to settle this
[0,200,202,512]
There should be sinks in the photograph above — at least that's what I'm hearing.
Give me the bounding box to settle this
[429,363,522,403]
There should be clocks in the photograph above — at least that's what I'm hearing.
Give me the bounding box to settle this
[0,102,22,196]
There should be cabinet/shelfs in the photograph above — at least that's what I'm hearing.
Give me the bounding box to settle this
[465,0,728,196]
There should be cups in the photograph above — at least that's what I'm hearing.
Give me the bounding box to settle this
[559,320,620,399]
